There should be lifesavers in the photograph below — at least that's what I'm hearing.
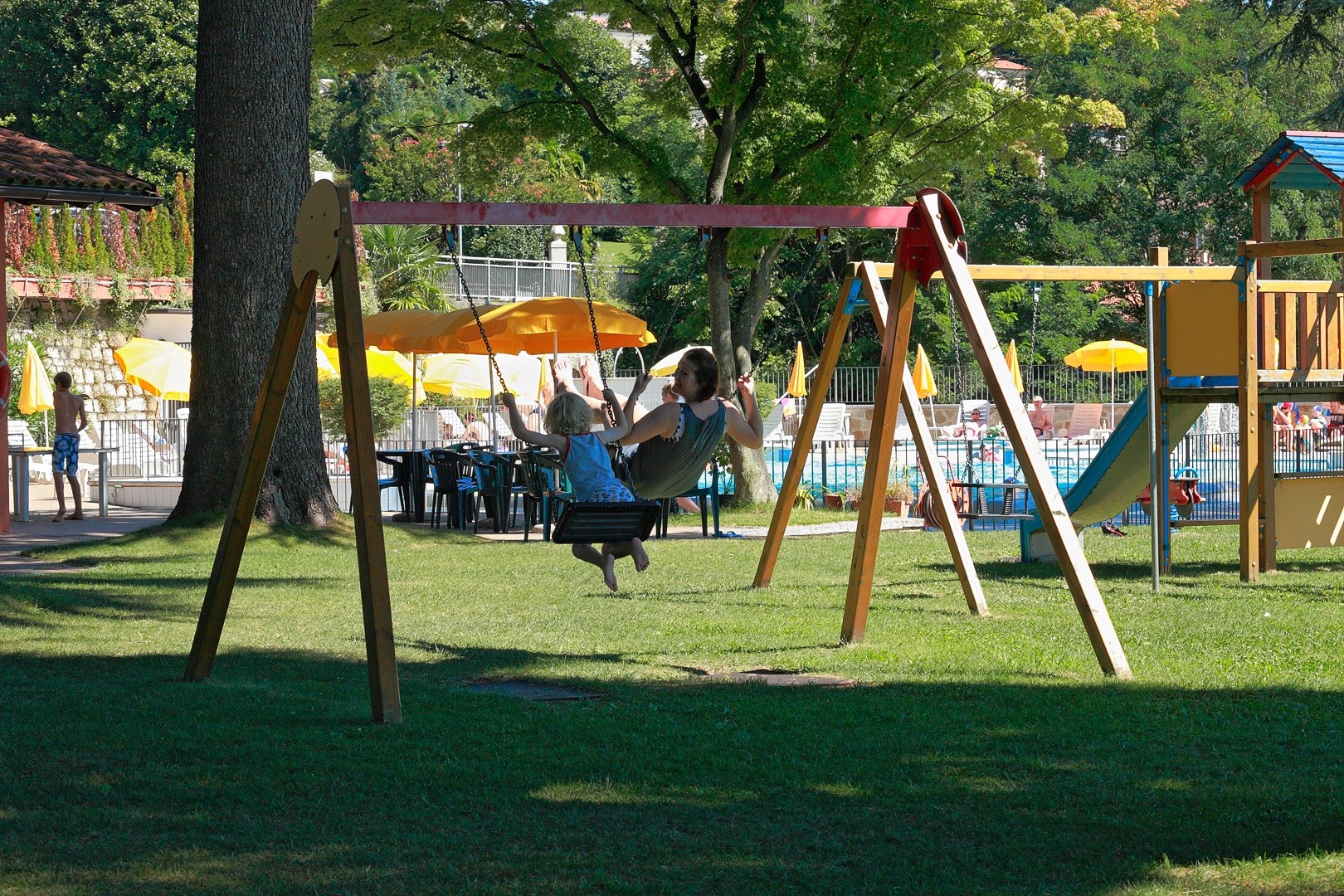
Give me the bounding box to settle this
[0,350,11,415]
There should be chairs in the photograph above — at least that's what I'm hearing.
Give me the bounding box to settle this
[1028,404,1055,438]
[343,442,576,541]
[8,420,52,484]
[75,420,169,498]
[439,410,466,439]
[1064,404,1103,445]
[941,399,990,440]
[763,404,785,444]
[482,413,512,439]
[653,455,719,538]
[796,403,852,448]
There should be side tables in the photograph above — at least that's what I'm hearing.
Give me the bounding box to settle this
[1092,429,1114,439]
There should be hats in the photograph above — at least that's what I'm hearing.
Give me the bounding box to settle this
[1281,402,1293,409]
[1033,396,1042,401]
[1314,405,1321,412]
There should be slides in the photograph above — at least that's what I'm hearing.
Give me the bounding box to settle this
[1019,380,1210,566]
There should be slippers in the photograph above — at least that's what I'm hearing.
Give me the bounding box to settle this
[725,531,742,536]
[712,530,730,538]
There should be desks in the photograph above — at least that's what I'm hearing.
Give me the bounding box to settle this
[928,427,943,440]
[8,447,119,521]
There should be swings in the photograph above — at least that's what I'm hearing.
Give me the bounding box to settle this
[444,229,661,544]
[944,288,1039,520]
[635,233,827,465]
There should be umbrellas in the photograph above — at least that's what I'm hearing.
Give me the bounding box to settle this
[113,337,191,417]
[1005,339,1024,396]
[913,343,938,427]
[18,341,55,449]
[1064,338,1148,428]
[316,292,712,433]
[787,341,807,426]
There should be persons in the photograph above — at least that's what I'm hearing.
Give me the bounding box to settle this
[1027,396,1052,436]
[979,443,1001,462]
[552,348,764,502]
[502,388,650,592]
[444,381,701,514]
[52,372,89,521]
[942,408,987,439]
[1273,402,1344,455]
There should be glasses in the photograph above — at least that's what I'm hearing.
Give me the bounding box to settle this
[972,413,978,415]
